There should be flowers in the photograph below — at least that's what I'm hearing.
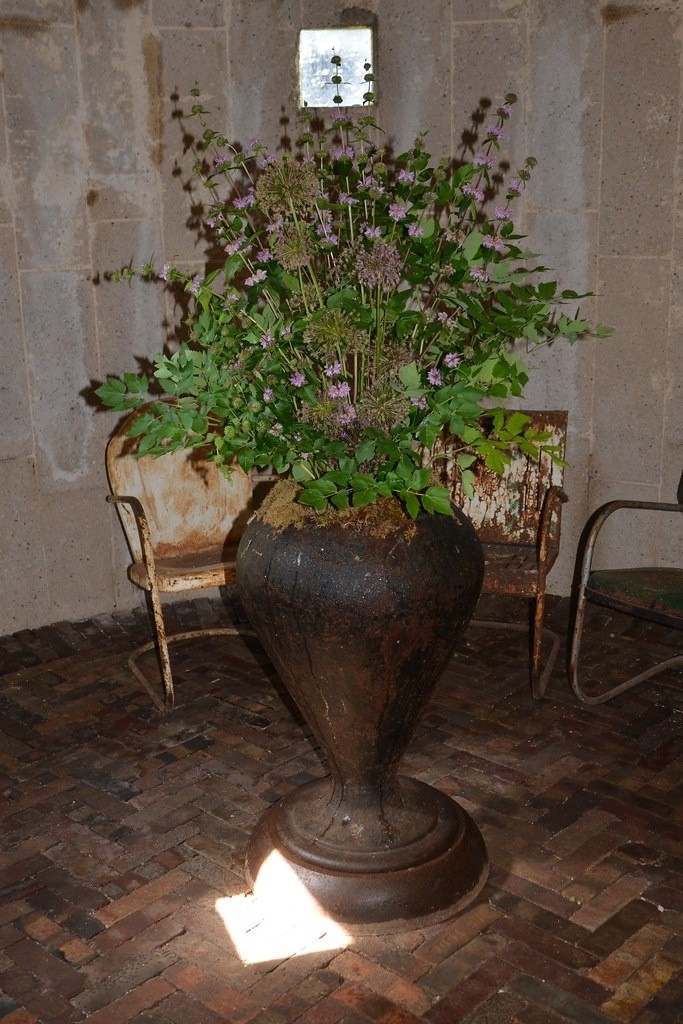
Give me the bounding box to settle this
[89,64,611,511]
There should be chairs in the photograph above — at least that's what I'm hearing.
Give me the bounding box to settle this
[108,399,257,711]
[573,470,683,703]
[421,407,566,702]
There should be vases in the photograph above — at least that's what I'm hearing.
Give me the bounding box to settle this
[231,466,492,930]
